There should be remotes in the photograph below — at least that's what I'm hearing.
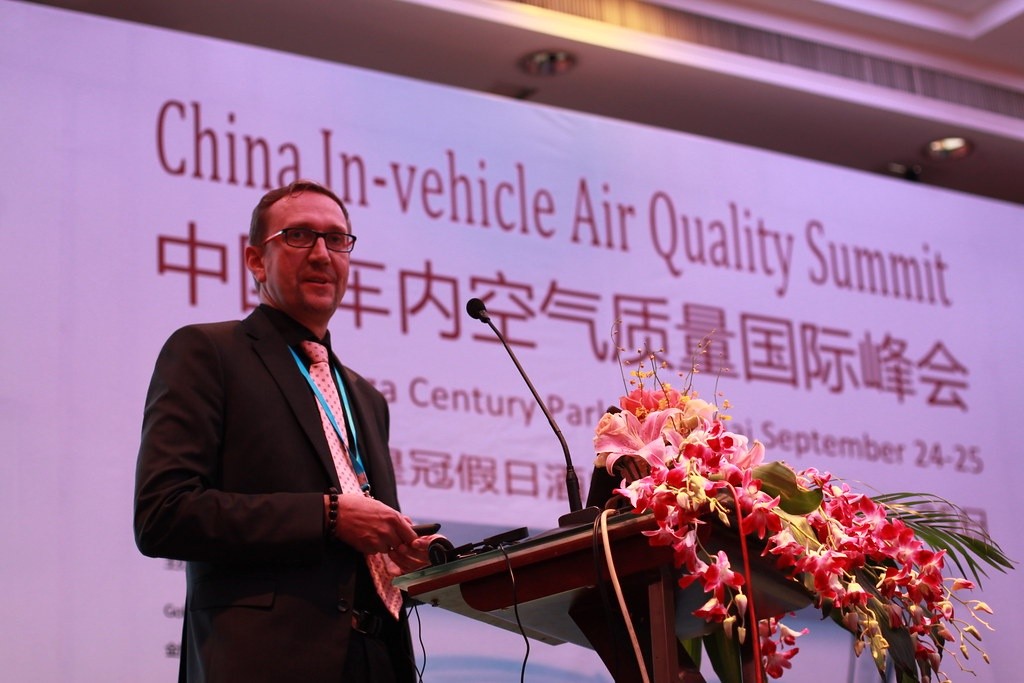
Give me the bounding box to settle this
[412,523,442,537]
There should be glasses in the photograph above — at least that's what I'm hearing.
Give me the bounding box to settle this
[257,228,358,253]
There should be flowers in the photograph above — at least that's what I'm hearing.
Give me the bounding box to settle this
[592,316,1020,683]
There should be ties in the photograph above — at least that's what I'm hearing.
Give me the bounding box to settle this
[299,339,404,623]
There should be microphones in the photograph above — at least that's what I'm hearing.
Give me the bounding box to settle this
[467,298,600,526]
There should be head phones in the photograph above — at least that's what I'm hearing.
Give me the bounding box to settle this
[428,527,530,565]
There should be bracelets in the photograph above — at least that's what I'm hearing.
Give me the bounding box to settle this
[323,487,338,543]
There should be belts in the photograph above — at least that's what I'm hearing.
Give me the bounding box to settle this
[350,607,386,636]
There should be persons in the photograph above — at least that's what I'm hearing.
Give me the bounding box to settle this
[133,180,448,683]
